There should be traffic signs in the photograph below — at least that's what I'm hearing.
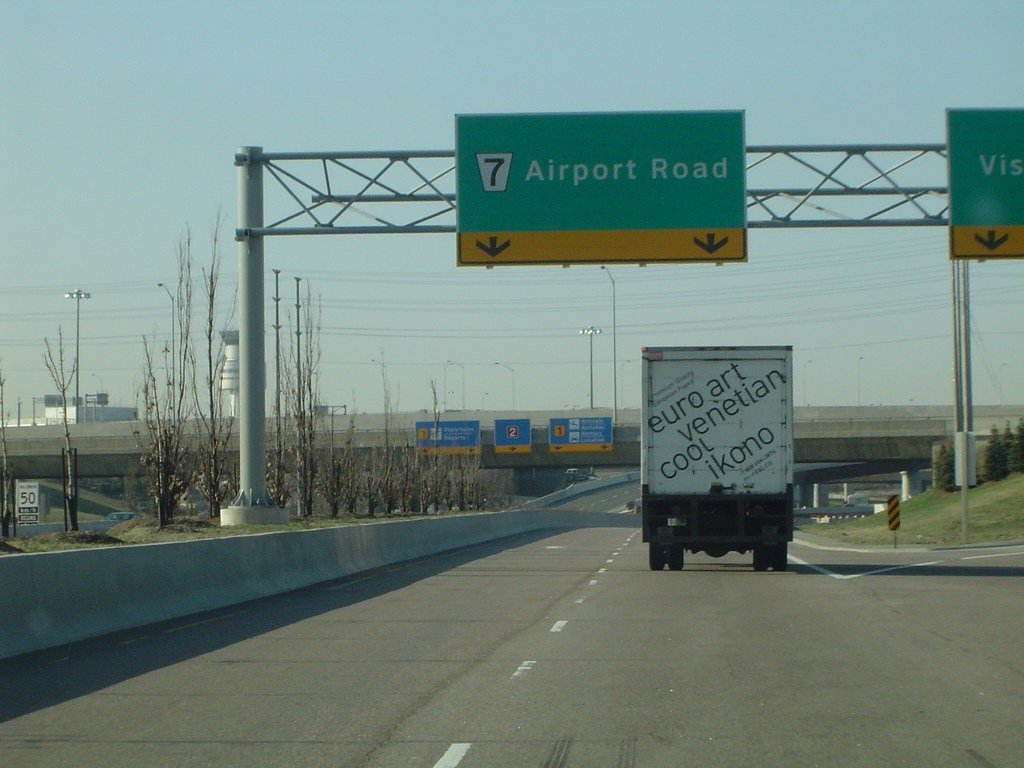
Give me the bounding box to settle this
[548,416,614,453]
[453,109,748,267]
[494,419,532,453]
[945,104,1024,261]
[414,420,481,456]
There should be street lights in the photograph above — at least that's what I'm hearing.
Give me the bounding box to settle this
[447,360,467,412]
[369,358,390,409]
[89,372,104,424]
[159,282,176,421]
[600,265,619,427]
[63,288,94,425]
[580,326,601,410]
[494,362,516,409]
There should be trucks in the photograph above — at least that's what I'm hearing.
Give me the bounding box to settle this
[637,344,794,571]
